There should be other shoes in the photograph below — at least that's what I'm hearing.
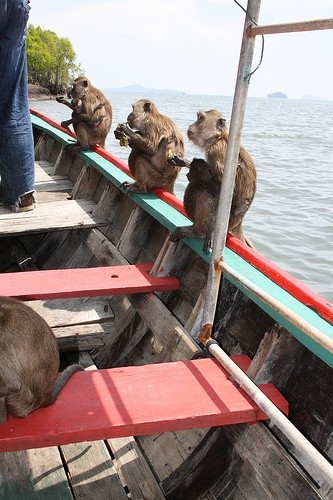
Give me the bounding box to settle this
[17,194,34,212]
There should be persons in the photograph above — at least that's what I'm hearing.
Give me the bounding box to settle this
[0,0,37,212]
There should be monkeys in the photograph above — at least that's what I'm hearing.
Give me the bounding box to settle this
[56,77,112,153]
[119,99,185,197]
[169,110,257,255]
[0,297,85,425]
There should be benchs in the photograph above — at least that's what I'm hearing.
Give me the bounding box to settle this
[0,356,289,454]
[0,261,180,302]
